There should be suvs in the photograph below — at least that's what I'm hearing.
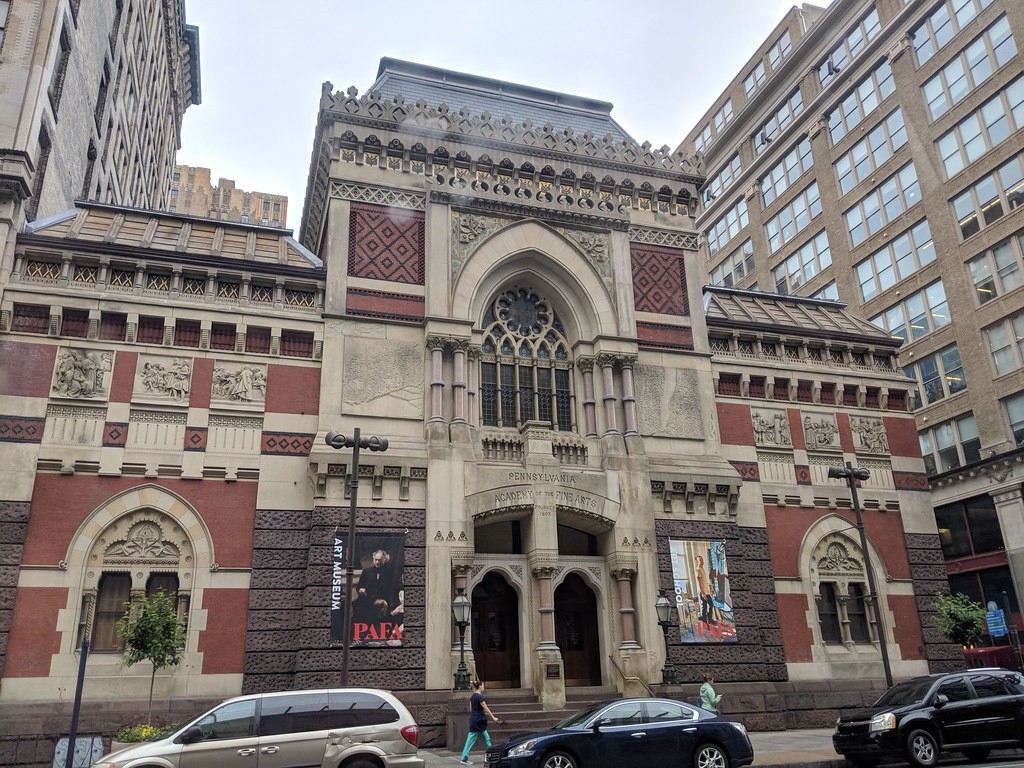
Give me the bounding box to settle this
[88,689,425,768]
[833,668,1024,768]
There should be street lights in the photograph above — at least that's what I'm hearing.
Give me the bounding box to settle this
[827,461,894,691]
[325,428,388,688]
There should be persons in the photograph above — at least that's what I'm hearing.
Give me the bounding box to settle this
[460,681,499,765]
[699,673,724,715]
[694,555,717,629]
[352,549,395,622]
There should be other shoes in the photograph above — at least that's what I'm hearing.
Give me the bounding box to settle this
[460,760,474,765]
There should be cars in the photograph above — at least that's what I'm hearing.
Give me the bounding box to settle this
[484,697,754,768]
